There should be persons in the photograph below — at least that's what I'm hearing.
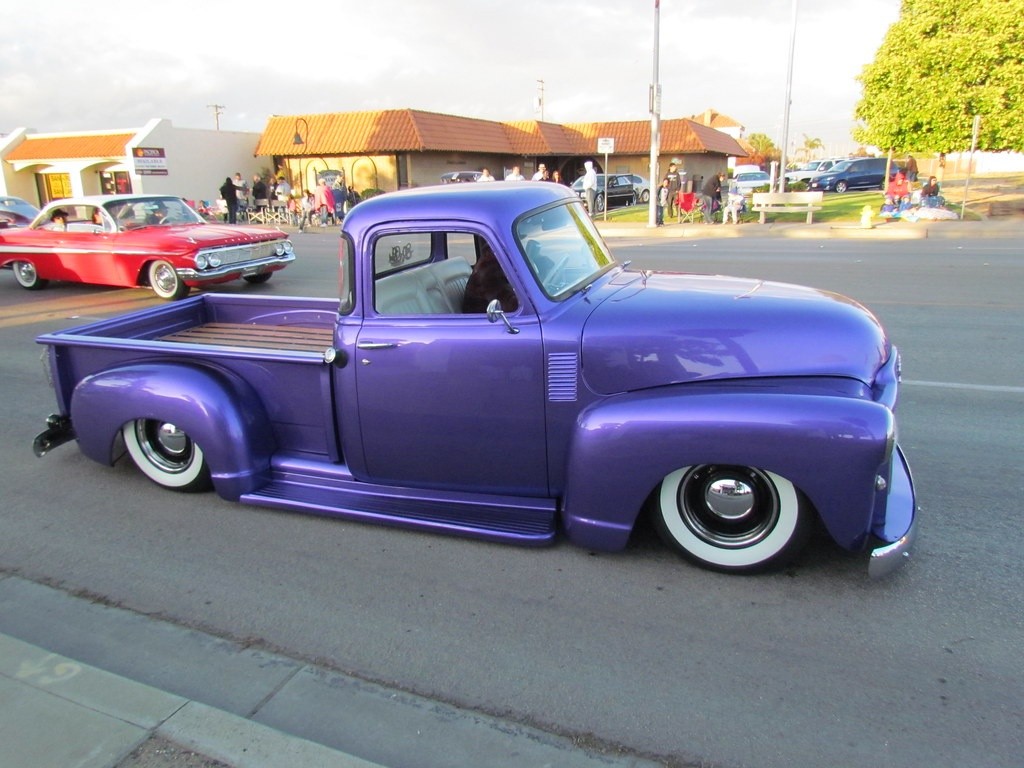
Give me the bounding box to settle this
[582,161,597,217]
[477,168,495,182]
[315,175,361,227]
[143,200,170,225]
[90,206,122,233]
[219,173,249,225]
[181,197,207,221]
[298,189,315,233]
[700,172,745,226]
[531,163,565,185]
[50,208,69,232]
[461,224,528,316]
[884,156,938,210]
[655,163,681,228]
[505,166,526,181]
[268,176,297,226]
[252,175,267,223]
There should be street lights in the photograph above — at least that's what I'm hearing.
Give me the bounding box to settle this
[293,118,309,196]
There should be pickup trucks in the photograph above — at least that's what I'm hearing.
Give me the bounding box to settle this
[30,182,920,580]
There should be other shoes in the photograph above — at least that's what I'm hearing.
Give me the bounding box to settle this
[588,212,594,216]
[320,222,328,228]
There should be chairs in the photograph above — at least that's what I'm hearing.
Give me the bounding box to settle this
[676,192,745,224]
[216,198,332,226]
[121,206,140,229]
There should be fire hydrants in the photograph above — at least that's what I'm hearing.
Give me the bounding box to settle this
[860,206,876,229]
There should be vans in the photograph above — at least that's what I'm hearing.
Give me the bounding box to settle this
[809,156,900,193]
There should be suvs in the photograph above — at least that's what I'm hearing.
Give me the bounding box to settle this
[778,159,852,190]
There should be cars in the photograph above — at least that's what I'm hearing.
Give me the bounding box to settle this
[0,195,52,228]
[0,195,297,300]
[570,173,638,212]
[614,174,651,202]
[729,165,770,194]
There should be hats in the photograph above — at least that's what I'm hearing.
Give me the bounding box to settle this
[50,209,68,221]
[895,173,905,181]
[907,154,913,158]
[151,200,170,212]
[538,164,547,169]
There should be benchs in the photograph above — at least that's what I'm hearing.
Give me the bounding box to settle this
[752,191,823,224]
[349,256,474,315]
